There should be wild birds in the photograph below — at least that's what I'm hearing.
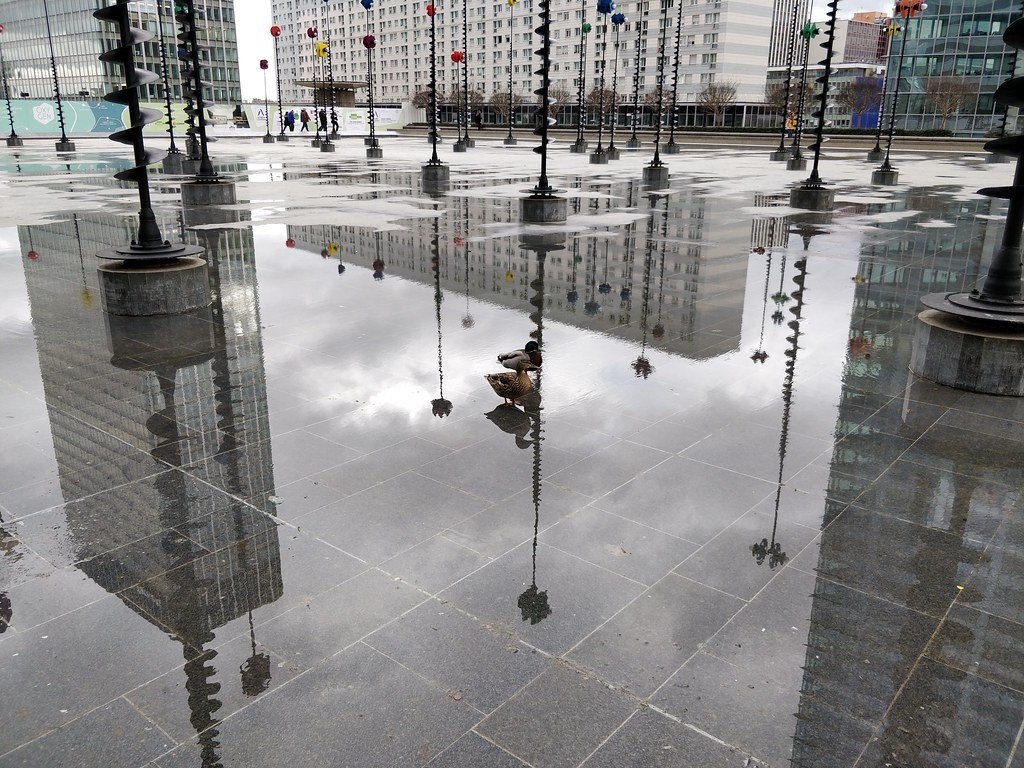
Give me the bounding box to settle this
[497,341,546,370]
[511,391,545,440]
[485,405,538,449]
[483,359,542,408]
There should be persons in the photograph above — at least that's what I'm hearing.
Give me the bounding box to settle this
[475,110,482,131]
[283,107,339,132]
[784,112,797,138]
